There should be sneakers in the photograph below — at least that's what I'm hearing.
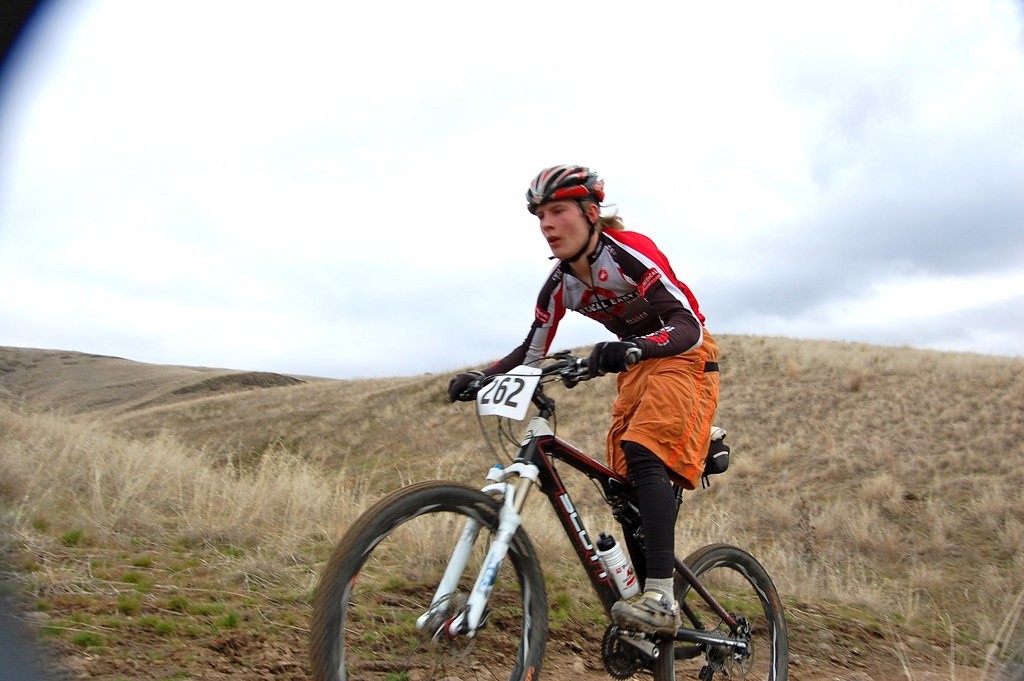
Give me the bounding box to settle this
[611,588,681,636]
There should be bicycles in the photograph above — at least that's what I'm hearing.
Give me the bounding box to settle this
[309,348,787,681]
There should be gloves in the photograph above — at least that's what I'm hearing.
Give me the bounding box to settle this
[446,370,487,403]
[588,342,642,376]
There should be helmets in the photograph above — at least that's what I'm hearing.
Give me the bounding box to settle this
[526,165,606,213]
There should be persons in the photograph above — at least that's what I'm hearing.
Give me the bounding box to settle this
[448,165,720,635]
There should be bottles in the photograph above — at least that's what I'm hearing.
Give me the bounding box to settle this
[596,531,640,601]
